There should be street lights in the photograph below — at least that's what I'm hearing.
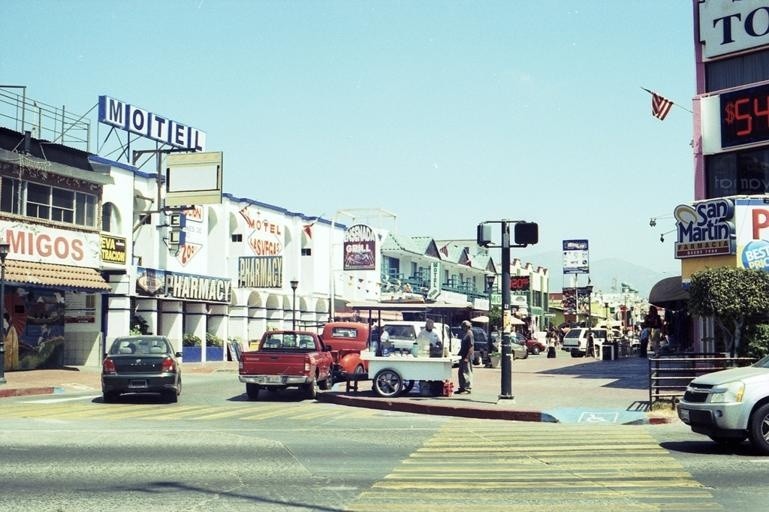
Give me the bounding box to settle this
[288,276,300,332]
[0,236,10,384]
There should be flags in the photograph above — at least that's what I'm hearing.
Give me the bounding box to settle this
[649,88,674,122]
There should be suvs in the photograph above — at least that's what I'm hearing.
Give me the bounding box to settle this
[676,353,769,455]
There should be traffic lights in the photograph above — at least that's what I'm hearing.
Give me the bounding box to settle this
[476,223,492,247]
[514,221,539,246]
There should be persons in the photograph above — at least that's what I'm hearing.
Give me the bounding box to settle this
[545,334,551,353]
[547,333,560,358]
[659,333,668,344]
[452,319,475,394]
[584,332,596,358]
[416,318,442,356]
[638,322,651,358]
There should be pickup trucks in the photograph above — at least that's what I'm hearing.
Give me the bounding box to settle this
[237,329,336,400]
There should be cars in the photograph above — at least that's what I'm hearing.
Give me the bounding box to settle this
[320,321,371,382]
[367,314,644,367]
[99,333,186,405]
